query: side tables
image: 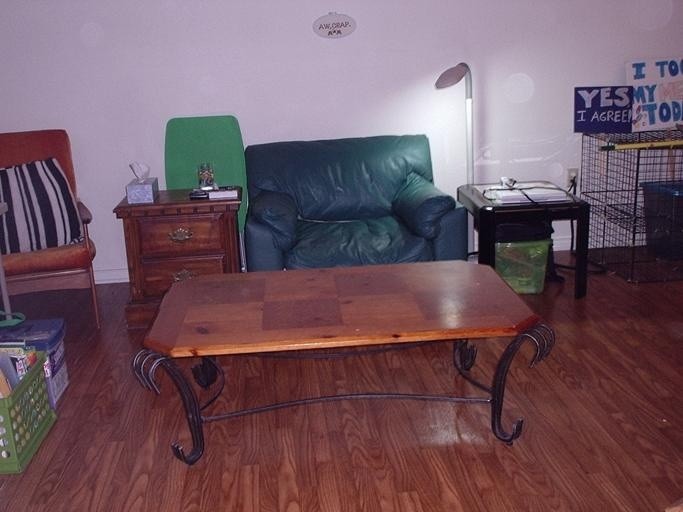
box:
[453,179,592,299]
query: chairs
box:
[243,131,470,271]
[0,128,103,334]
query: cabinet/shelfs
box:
[110,188,242,329]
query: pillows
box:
[0,157,85,255]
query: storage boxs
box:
[494,238,553,295]
[0,315,72,406]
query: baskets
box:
[0,348,58,475]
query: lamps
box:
[434,60,479,262]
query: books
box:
[0,340,50,458]
